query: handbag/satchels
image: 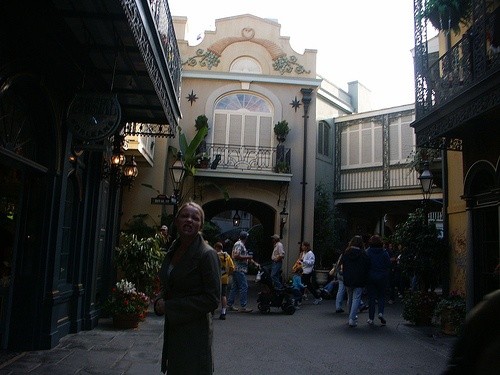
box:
[292,262,303,273]
[329,266,337,276]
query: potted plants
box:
[401,291,437,326]
[437,293,466,336]
[275,162,288,173]
[195,115,209,155]
[273,120,289,166]
[196,152,211,169]
[419,0,473,37]
[113,232,170,321]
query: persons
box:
[271,234,286,288]
[155,202,221,375]
[333,234,404,327]
[299,241,322,305]
[293,267,307,307]
[157,225,262,319]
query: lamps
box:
[122,156,139,193]
[417,161,435,199]
[169,151,189,205]
[233,210,240,227]
[104,145,126,174]
[68,139,89,164]
[279,208,288,225]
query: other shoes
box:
[314,297,322,304]
[220,314,226,320]
[349,319,357,327]
[336,310,344,313]
[367,319,374,324]
[240,307,252,313]
[389,300,395,304]
[399,296,403,299]
[227,305,239,311]
[378,313,386,323]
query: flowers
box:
[106,278,151,330]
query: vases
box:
[315,270,329,288]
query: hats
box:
[271,234,280,241]
[160,225,168,229]
[240,231,249,237]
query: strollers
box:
[255,263,306,315]
